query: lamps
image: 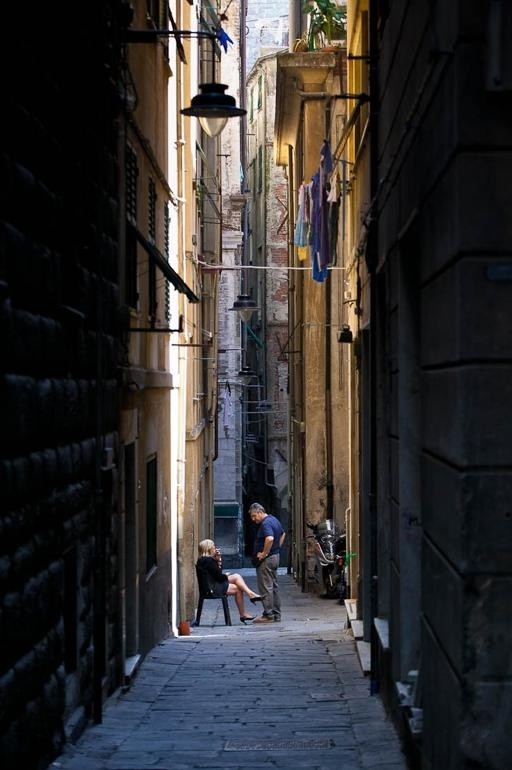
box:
[123,24,248,137]
[197,253,261,322]
[218,346,257,386]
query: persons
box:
[249,503,286,624]
[196,538,266,626]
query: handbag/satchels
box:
[251,557,263,568]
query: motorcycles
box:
[304,515,346,599]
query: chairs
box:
[193,563,232,626]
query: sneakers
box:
[254,616,280,624]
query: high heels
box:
[249,594,266,606]
[240,614,257,625]
